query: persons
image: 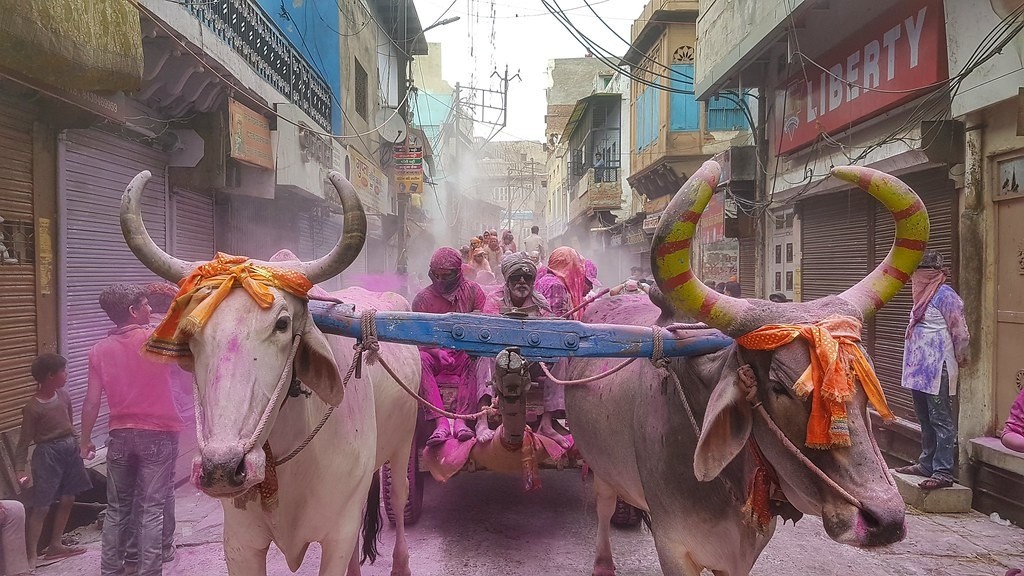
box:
[895,248,973,488]
[459,227,516,282]
[409,248,488,449]
[525,227,547,268]
[80,284,179,576]
[121,285,183,572]
[0,500,35,576]
[533,246,583,377]
[610,265,794,309]
[576,257,603,313]
[14,354,93,576]
[1001,387,1023,451]
[473,252,572,447]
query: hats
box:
[918,249,943,269]
[429,246,463,269]
[470,229,515,264]
[501,251,537,281]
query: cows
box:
[556,157,933,575]
[118,170,418,576]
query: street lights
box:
[399,15,461,130]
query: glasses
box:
[509,274,533,282]
[433,273,453,280]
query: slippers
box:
[427,427,453,446]
[455,427,476,440]
[918,479,953,488]
[896,465,928,476]
[45,545,86,560]
